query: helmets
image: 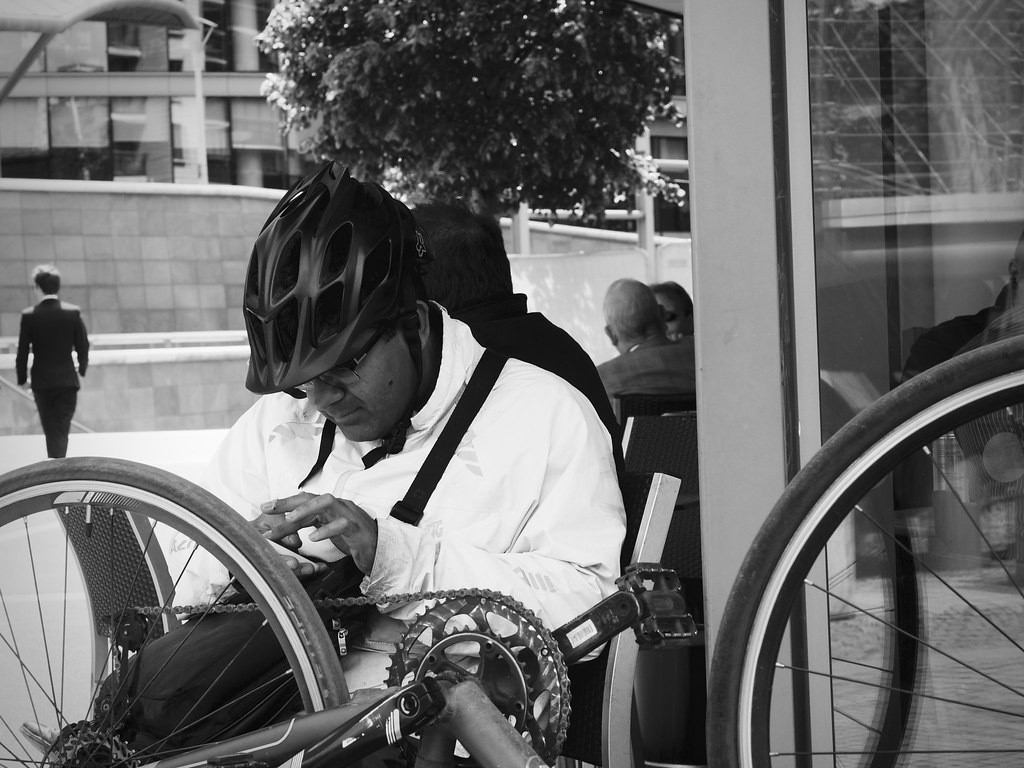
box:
[242,160,434,396]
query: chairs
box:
[53,502,199,723]
[622,414,707,649]
[455,472,684,768]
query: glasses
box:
[297,325,387,389]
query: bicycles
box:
[1,334,1024,768]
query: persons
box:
[410,199,635,577]
[596,278,696,417]
[649,281,694,343]
[900,227,1023,593]
[15,264,90,459]
[184,161,628,768]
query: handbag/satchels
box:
[96,555,377,763]
